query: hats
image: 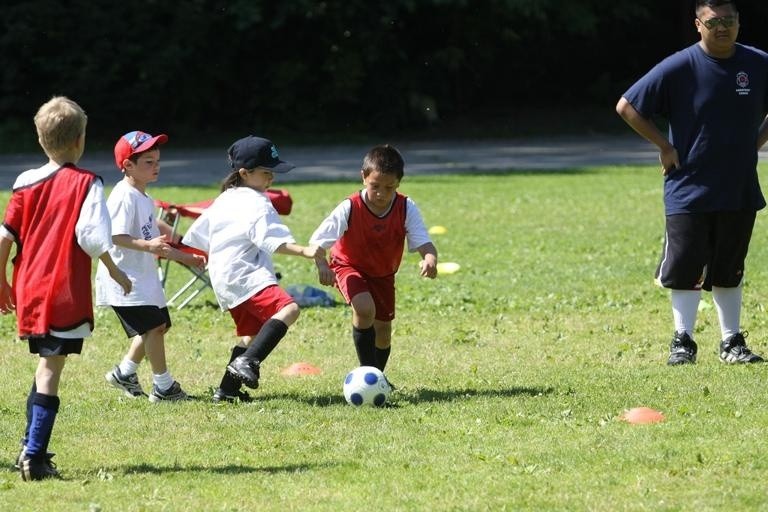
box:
[114,131,168,173]
[228,135,295,173]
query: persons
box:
[181,134,326,404]
[0,94,132,482]
[615,1,768,366]
[96,131,207,404]
[309,144,437,392]
[155,219,187,245]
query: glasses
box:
[697,14,737,28]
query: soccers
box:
[343,366,388,410]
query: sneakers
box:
[20,454,65,481]
[667,331,697,365]
[719,331,763,363]
[105,357,259,403]
